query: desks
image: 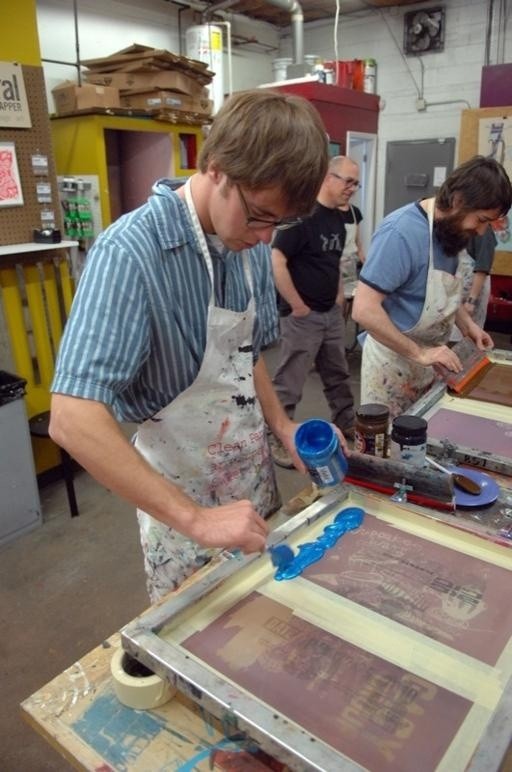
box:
[17,348,511,772]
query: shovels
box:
[424,455,485,495]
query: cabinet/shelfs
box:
[49,110,209,240]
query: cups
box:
[293,418,349,487]
[314,69,334,85]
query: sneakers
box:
[266,422,296,469]
[338,424,357,442]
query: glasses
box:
[332,172,363,191]
[237,189,304,232]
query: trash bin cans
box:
[0,370,44,545]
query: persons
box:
[337,201,365,324]
[47,89,351,603]
[263,154,360,470]
[351,155,512,426]
[450,226,498,347]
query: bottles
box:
[335,58,362,92]
[363,56,377,97]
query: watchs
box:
[466,296,480,307]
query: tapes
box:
[41,229,52,236]
[109,646,178,710]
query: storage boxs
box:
[50,69,216,117]
